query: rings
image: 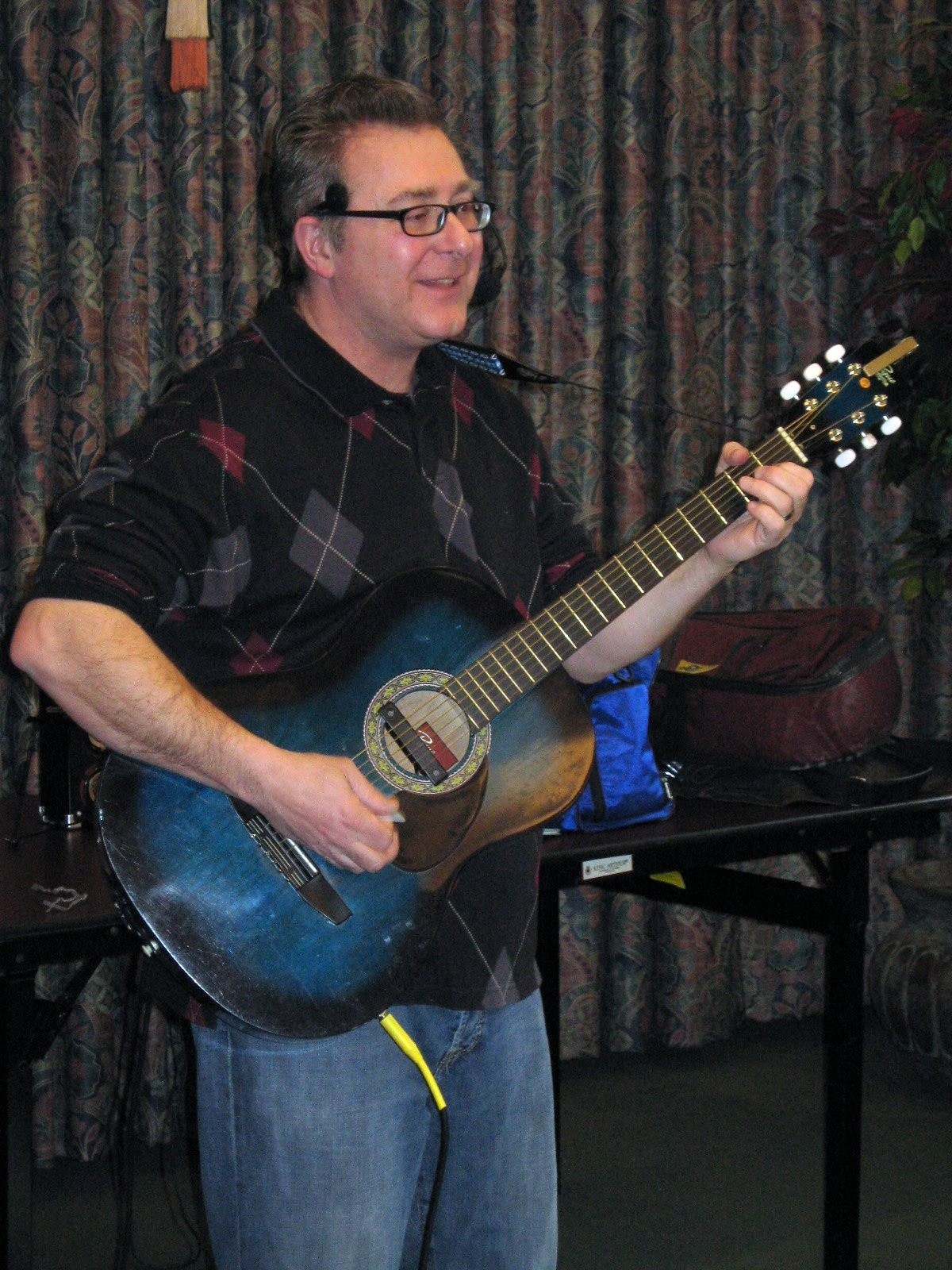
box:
[782,506,795,522]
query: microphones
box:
[475,221,508,303]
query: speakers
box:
[39,696,109,831]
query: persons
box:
[9,73,814,1270]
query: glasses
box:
[307,197,493,237]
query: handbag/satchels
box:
[540,644,675,834]
[647,604,906,776]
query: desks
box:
[0,739,952,1270]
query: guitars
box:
[95,336,938,1045]
